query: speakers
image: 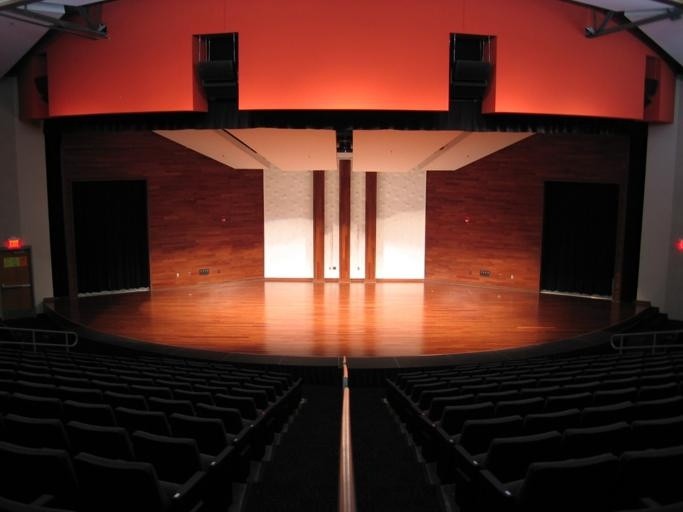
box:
[35,75,48,103]
[645,79,657,97]
[450,81,487,96]
[197,61,235,81]
[453,60,492,81]
[204,83,238,101]
